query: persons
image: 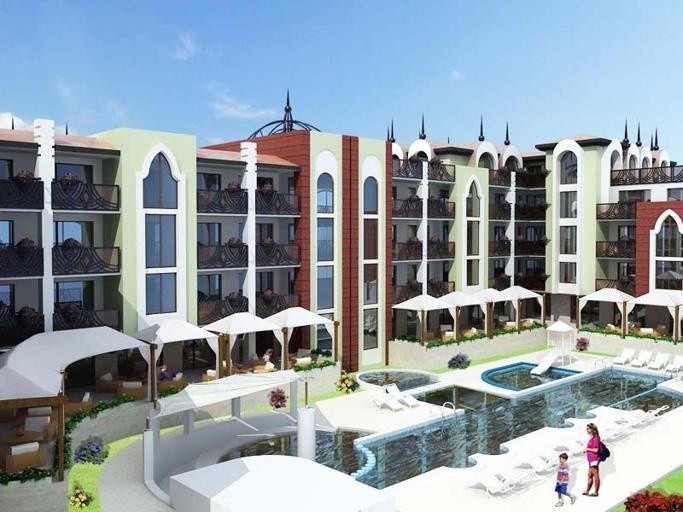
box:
[262,347,274,361]
[582,423,610,496]
[553,452,577,508]
[159,362,182,381]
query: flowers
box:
[266,370,360,410]
[575,335,591,351]
[449,353,471,370]
[68,434,110,508]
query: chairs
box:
[469,404,671,496]
[612,348,682,377]
[157,365,216,391]
[369,382,419,410]
[606,321,667,333]
[223,348,333,374]
[0,407,54,474]
[64,369,148,416]
[439,316,534,344]
